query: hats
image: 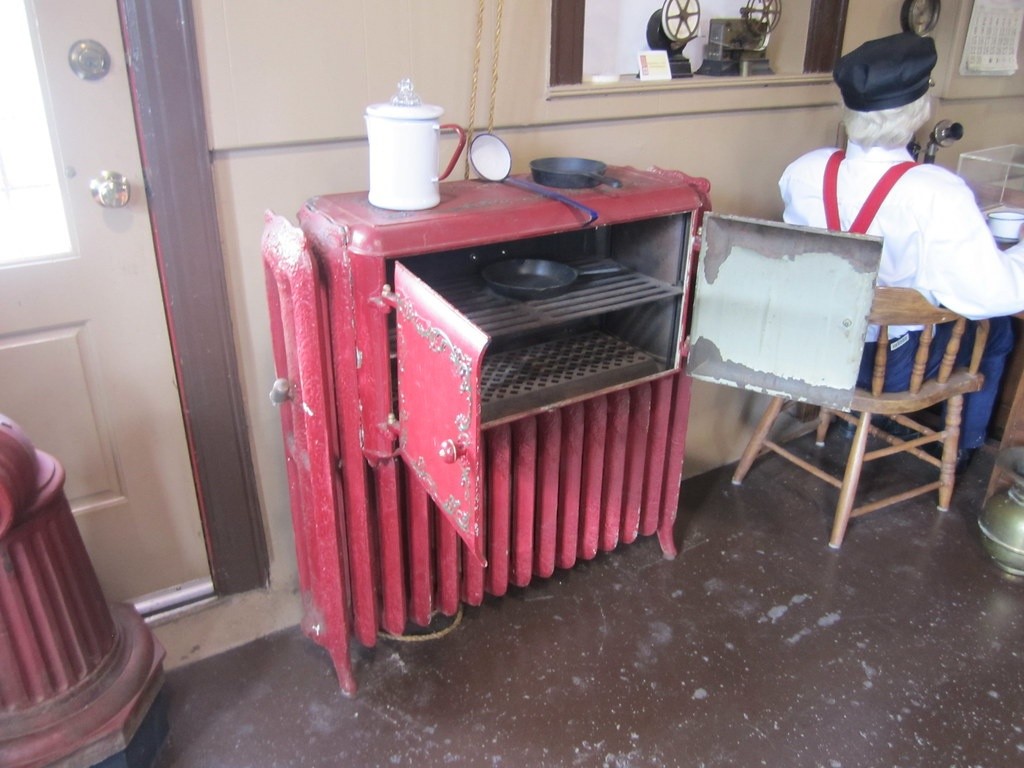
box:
[832,33,938,111]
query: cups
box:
[363,79,467,212]
[987,212,1024,238]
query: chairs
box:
[732,286,991,551]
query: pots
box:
[482,258,632,301]
[528,156,623,189]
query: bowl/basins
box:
[467,133,512,182]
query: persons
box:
[777,30,1024,468]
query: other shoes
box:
[839,413,921,439]
[932,441,975,474]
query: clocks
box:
[901,0,941,35]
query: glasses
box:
[928,78,935,87]
